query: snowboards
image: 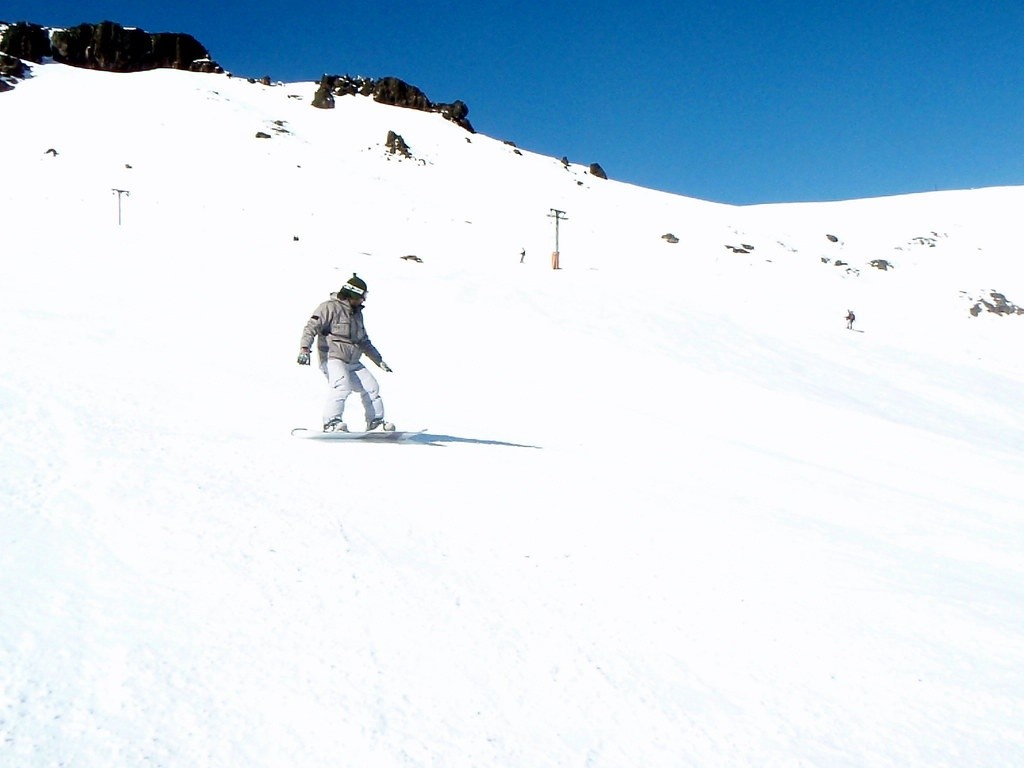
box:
[291,427,428,442]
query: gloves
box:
[379,362,393,373]
[297,352,310,365]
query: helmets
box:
[341,272,367,301]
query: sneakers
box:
[322,420,348,433]
[366,419,396,432]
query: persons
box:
[298,277,395,433]
